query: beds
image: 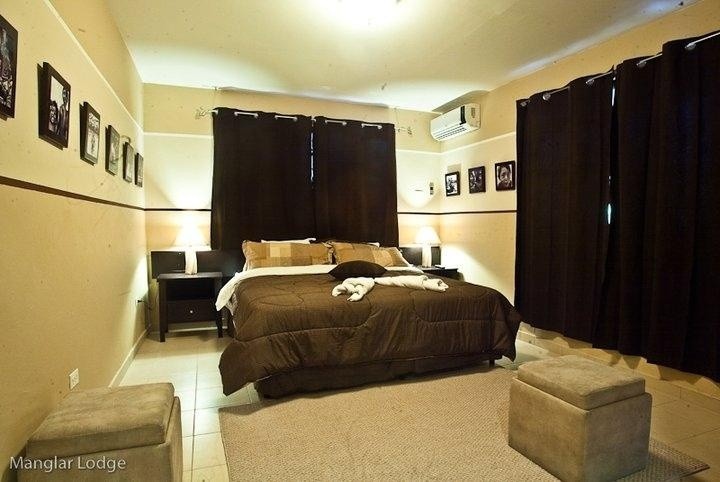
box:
[230,235,522,399]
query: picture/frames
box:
[0,14,19,118]
[37,58,145,187]
[444,170,460,197]
[467,166,487,194]
[493,161,516,191]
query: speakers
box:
[152,251,185,280]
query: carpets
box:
[216,362,708,482]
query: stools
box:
[17,383,186,482]
[505,355,655,482]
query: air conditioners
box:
[429,102,482,142]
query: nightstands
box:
[423,268,460,280]
[157,272,226,344]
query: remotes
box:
[435,264,445,270]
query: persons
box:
[498,164,512,188]
[0,28,15,105]
[49,98,59,133]
[446,177,457,194]
[469,169,483,191]
[58,87,69,139]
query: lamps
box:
[171,221,207,275]
[415,224,442,267]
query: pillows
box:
[240,237,408,273]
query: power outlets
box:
[135,297,143,307]
